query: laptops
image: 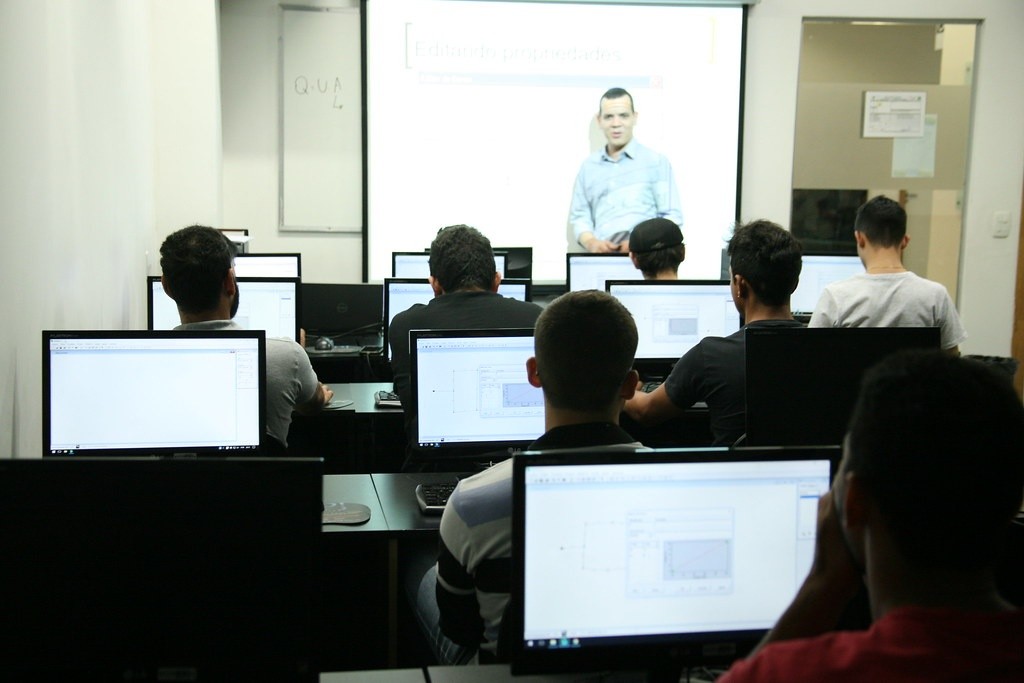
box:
[301,283,384,334]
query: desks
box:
[295,382,645,683]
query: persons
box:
[570,88,682,255]
[712,351,1024,683]
[628,217,686,281]
[399,289,699,666]
[807,195,967,356]
[389,224,544,473]
[159,225,333,455]
[621,222,807,447]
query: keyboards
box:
[415,483,458,514]
[374,391,401,407]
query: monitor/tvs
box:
[0,458,326,683]
[567,253,644,294]
[384,277,531,364]
[792,253,866,322]
[147,275,302,345]
[511,444,844,683]
[43,330,267,458]
[606,280,744,384]
[408,328,545,482]
[745,326,944,445]
[234,253,302,277]
[425,247,533,278]
[392,251,509,278]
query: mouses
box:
[315,336,334,350]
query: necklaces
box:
[868,266,904,269]
